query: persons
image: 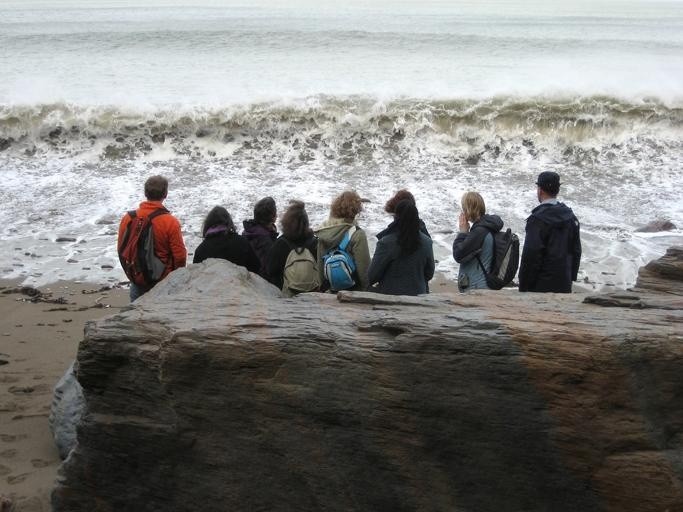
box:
[366,200,434,295]
[381,189,431,290]
[239,195,283,287]
[313,190,371,293]
[452,191,504,293]
[191,205,260,274]
[116,176,186,304]
[262,199,330,292]
[517,171,581,293]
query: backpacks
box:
[470,225,520,290]
[282,236,320,292]
[117,208,170,289]
[321,225,361,293]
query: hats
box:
[535,171,562,185]
